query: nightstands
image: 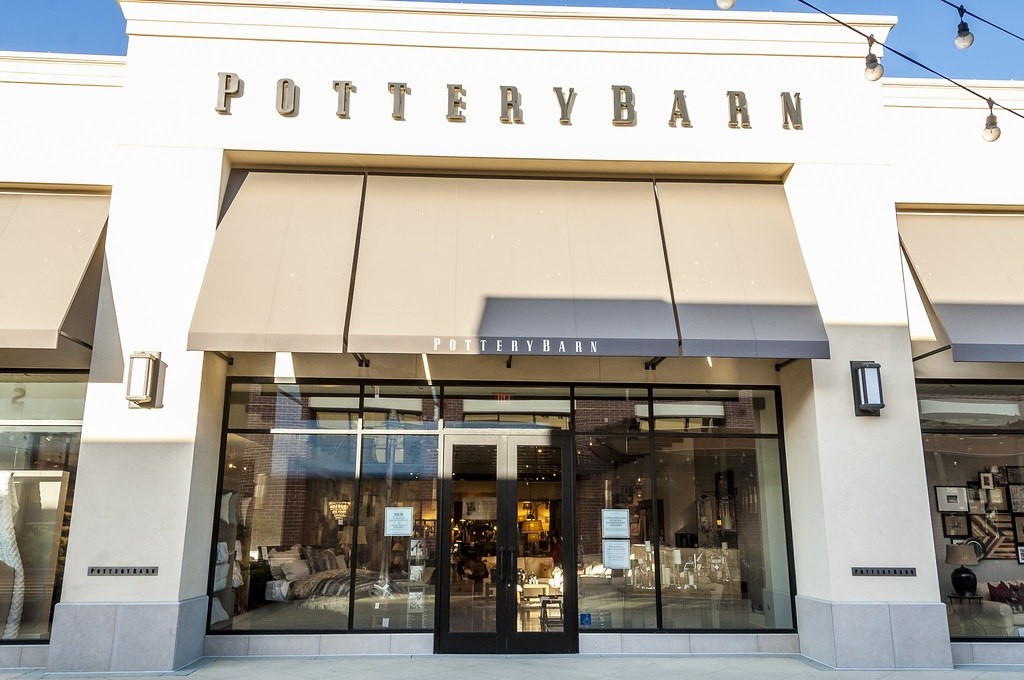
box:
[246,561,270,612]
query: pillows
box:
[220,491,256,527]
[577,560,608,576]
[538,563,554,578]
[267,544,348,583]
[986,581,1024,614]
[211,596,230,626]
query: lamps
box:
[849,360,885,417]
[392,543,405,564]
[339,526,368,544]
[716,493,722,526]
[413,497,551,558]
[623,539,732,592]
[945,544,979,597]
[124,350,162,409]
[329,501,351,526]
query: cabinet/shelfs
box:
[210,476,255,631]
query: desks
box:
[947,594,987,637]
[618,587,751,629]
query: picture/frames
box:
[408,592,423,610]
[601,509,630,538]
[408,538,426,558]
[602,539,631,570]
[933,464,1024,565]
[409,565,424,583]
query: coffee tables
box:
[522,583,549,597]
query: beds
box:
[258,544,409,623]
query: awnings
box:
[187,166,830,374]
[1,191,112,358]
[896,207,1024,368]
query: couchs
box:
[623,544,741,602]
[962,579,1024,638]
[575,552,613,587]
[481,556,564,596]
